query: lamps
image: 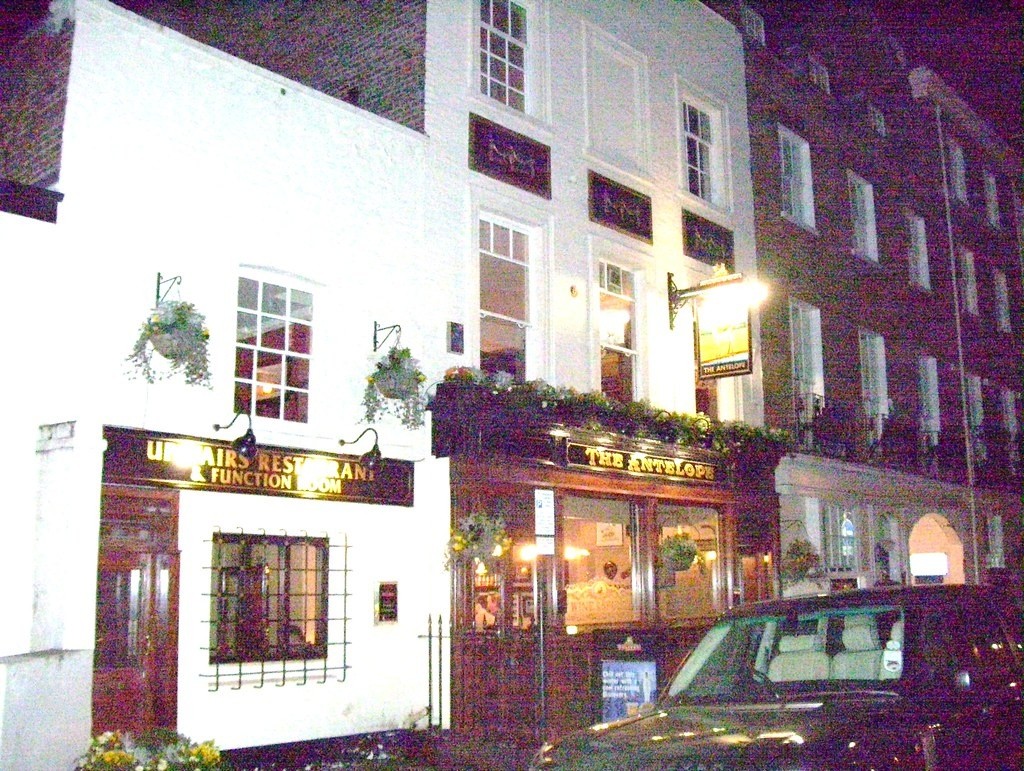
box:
[339,428,382,469]
[213,406,258,458]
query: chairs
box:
[829,613,884,680]
[879,620,904,680]
[767,636,830,680]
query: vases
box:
[150,334,186,359]
[377,380,417,399]
[664,557,692,571]
[458,546,492,559]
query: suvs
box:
[527,585,1023,771]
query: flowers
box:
[431,351,795,457]
[360,346,426,428]
[444,512,512,565]
[73,727,221,771]
[658,534,697,572]
[779,540,818,585]
[124,299,212,389]
